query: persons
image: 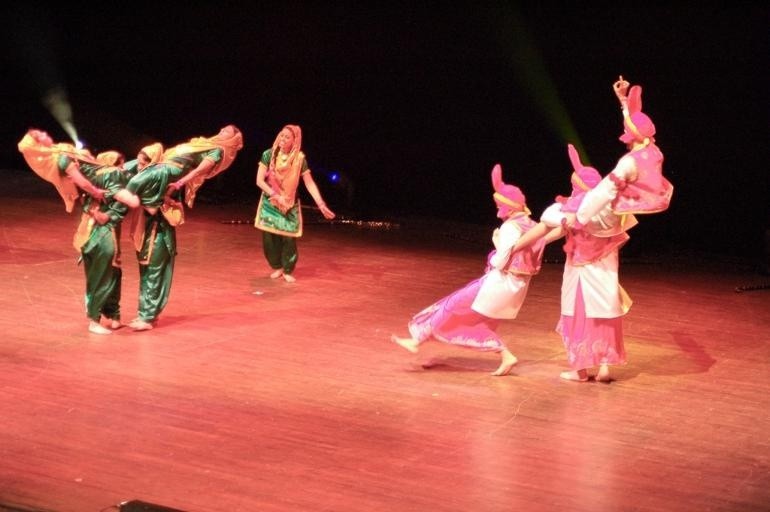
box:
[17,130,160,216]
[393,185,553,376]
[554,166,632,382]
[89,123,244,226]
[126,141,184,331]
[512,112,675,256]
[74,151,129,333]
[253,123,335,283]
